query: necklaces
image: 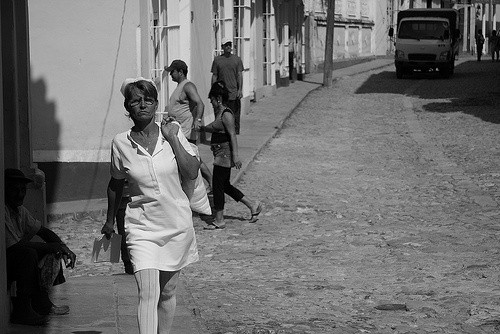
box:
[132,127,156,152]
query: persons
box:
[476,29,485,62]
[161,60,262,230]
[489,30,500,62]
[4,168,76,326]
[210,38,245,134]
[101,77,212,334]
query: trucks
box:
[389,8,460,79]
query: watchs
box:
[195,118,202,121]
